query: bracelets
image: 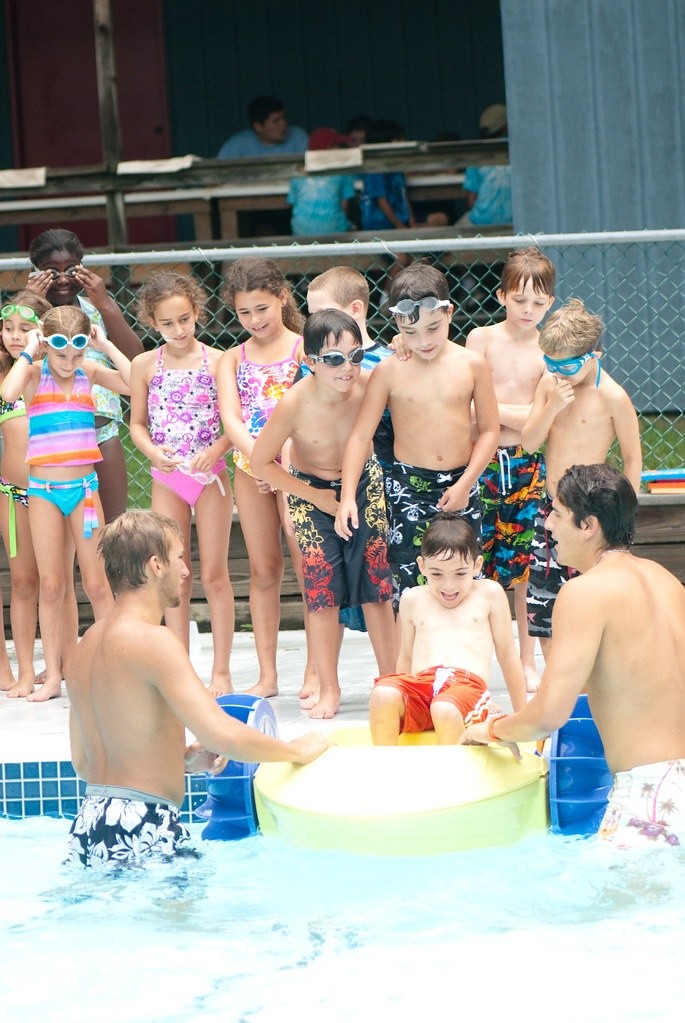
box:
[20,352,32,364]
[488,714,507,741]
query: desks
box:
[0,180,480,304]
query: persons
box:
[217,95,308,238]
[287,128,357,237]
[334,265,499,591]
[65,509,336,875]
[246,308,411,719]
[360,120,449,228]
[465,248,555,693]
[455,104,513,228]
[521,300,643,661]
[217,258,319,700]
[130,271,235,697]
[348,116,371,146]
[1,227,145,703]
[285,265,394,712]
[369,516,526,746]
[458,464,685,844]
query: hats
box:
[308,127,353,149]
[478,103,508,133]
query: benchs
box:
[0,238,513,295]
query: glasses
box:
[27,260,83,282]
[39,332,90,350]
[1,306,39,325]
[389,296,452,315]
[309,346,365,367]
[542,350,584,376]
